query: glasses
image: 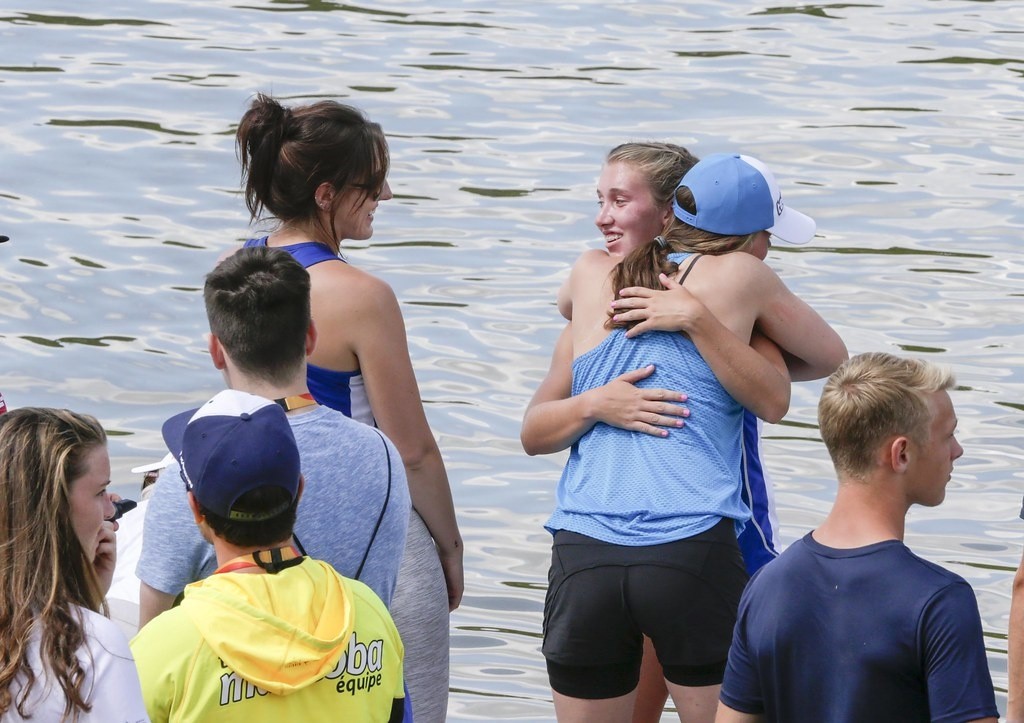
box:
[342,167,387,201]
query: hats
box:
[673,153,816,245]
[162,390,301,520]
[131,452,176,474]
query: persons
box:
[712,352,1001,723]
[135,247,410,632]
[0,406,149,723]
[129,389,408,723]
[520,142,849,722]
[1005,496,1024,723]
[237,95,463,723]
